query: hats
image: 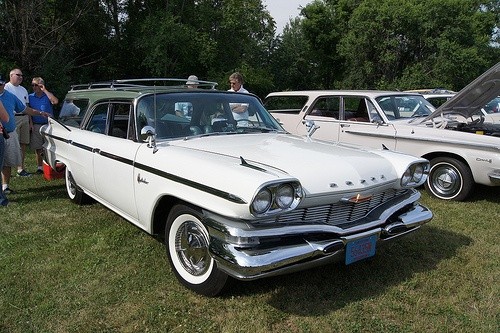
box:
[0,73,6,84]
[185,75,201,84]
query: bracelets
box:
[40,110,44,116]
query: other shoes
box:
[35,169,43,175]
[3,188,16,195]
[16,170,32,176]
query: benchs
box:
[312,111,396,122]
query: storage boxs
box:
[42,159,65,182]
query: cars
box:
[262,61,500,201]
[40,78,434,298]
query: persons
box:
[0,69,58,206]
[174,75,200,121]
[59,97,80,117]
[222,72,250,128]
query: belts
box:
[33,122,47,125]
[15,114,25,116]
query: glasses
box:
[0,84,3,86]
[31,84,39,86]
[16,74,24,77]
[230,82,235,85]
